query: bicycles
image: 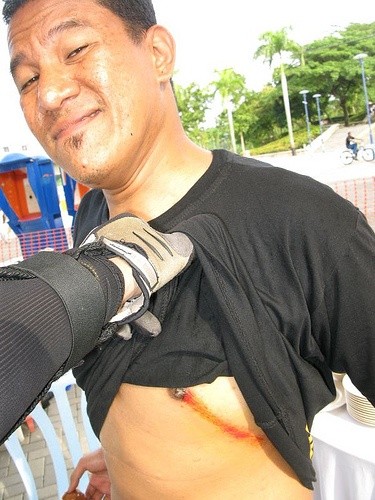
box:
[339,139,375,165]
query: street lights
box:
[352,53,373,144]
[300,90,312,151]
[313,94,324,145]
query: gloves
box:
[79,213,195,341]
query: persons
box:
[0,210,223,447]
[344,130,365,163]
[3,0,375,499]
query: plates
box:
[324,374,375,426]
